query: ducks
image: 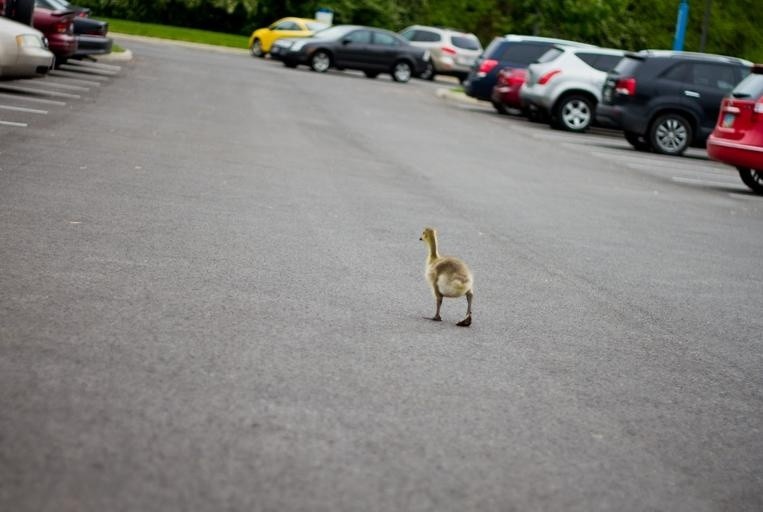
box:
[418,227,473,327]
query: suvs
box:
[525,44,625,132]
[462,34,579,114]
[397,23,483,80]
[596,46,752,155]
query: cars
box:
[248,16,331,57]
[492,66,527,114]
[704,63,763,193]
[269,25,430,83]
[0,0,113,82]
